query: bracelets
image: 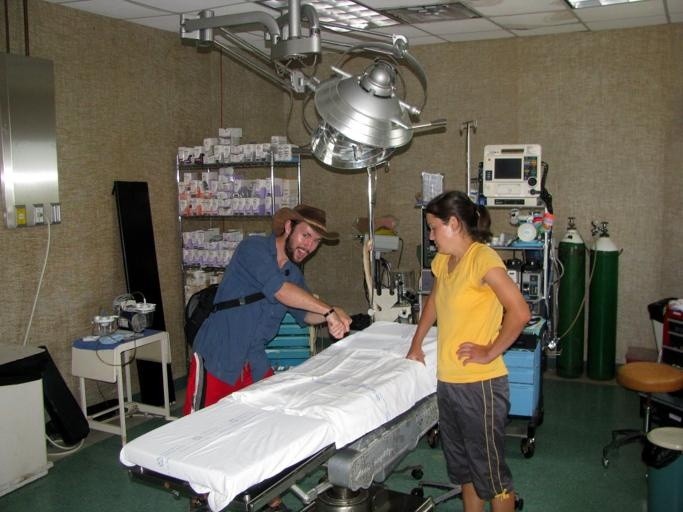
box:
[321,307,336,318]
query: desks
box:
[71,328,179,446]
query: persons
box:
[181,203,354,419]
[402,191,532,511]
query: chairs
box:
[648,297,679,358]
[185,281,220,368]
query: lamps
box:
[179,2,454,179]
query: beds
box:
[120,319,441,511]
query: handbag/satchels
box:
[183,283,218,347]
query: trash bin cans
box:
[646,427,683,512]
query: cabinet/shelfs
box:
[1,52,61,232]
[419,201,556,461]
[0,343,48,499]
[176,140,303,309]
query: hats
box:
[272,205,340,241]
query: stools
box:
[603,360,683,512]
